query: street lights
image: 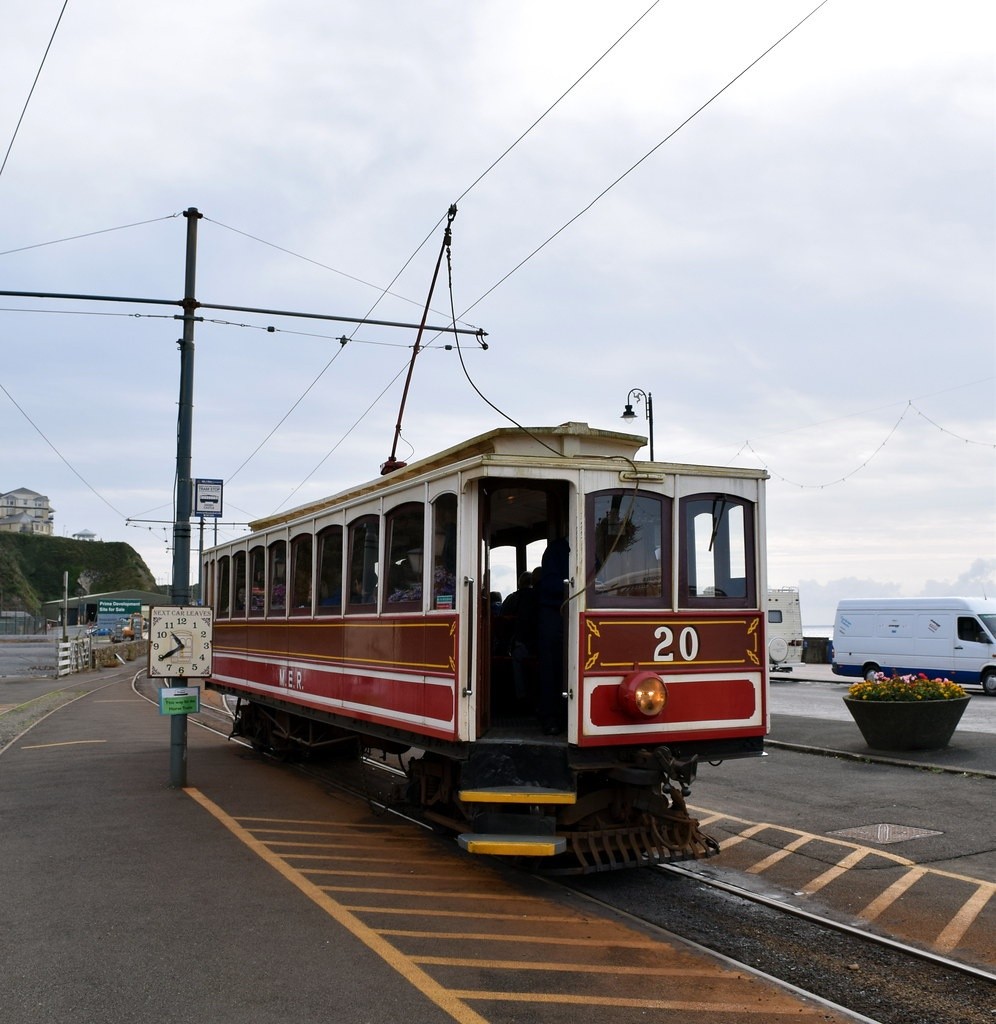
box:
[620,388,654,462]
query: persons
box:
[351,568,378,603]
[489,534,600,734]
[236,586,245,610]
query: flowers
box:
[850,670,967,701]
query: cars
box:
[86,628,114,636]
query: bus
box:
[199,419,771,880]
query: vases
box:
[842,694,974,751]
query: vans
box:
[831,596,996,698]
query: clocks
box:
[148,605,213,678]
